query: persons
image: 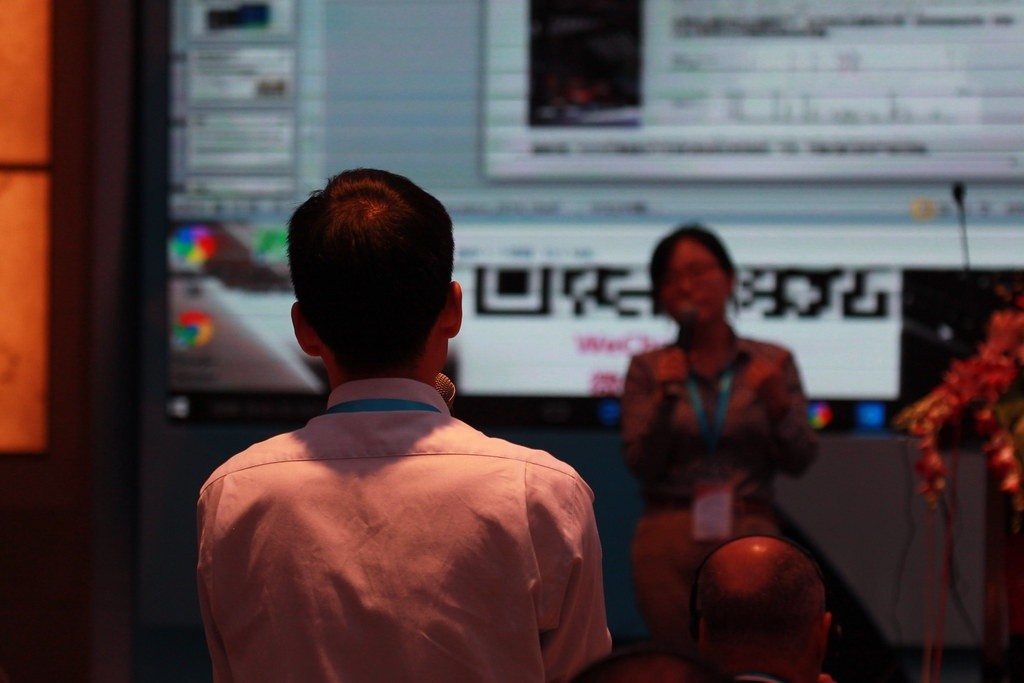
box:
[194,168,839,683]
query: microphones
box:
[434,373,457,416]
[952,182,970,271]
[663,305,698,400]
